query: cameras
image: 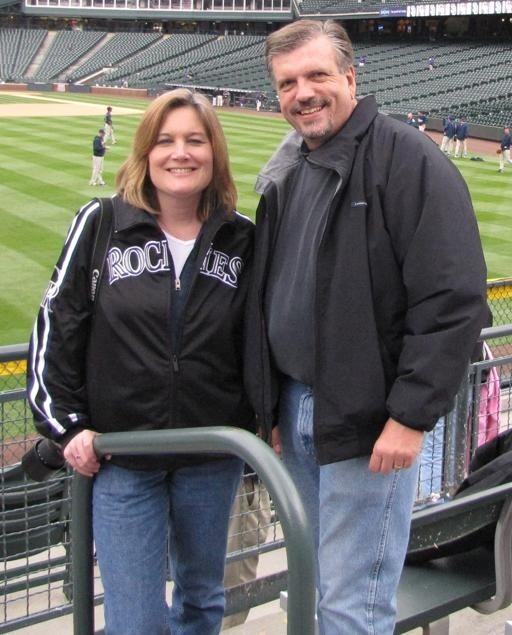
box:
[22,437,66,482]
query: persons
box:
[440,115,455,155]
[253,20,488,635]
[90,129,106,186]
[359,55,367,67]
[417,111,426,132]
[211,88,230,107]
[26,88,258,635]
[454,116,469,158]
[256,90,265,112]
[104,107,117,144]
[122,80,128,88]
[407,112,417,127]
[497,127,512,173]
[427,57,434,70]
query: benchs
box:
[279,482,512,635]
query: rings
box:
[74,455,80,460]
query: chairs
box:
[0,458,75,602]
